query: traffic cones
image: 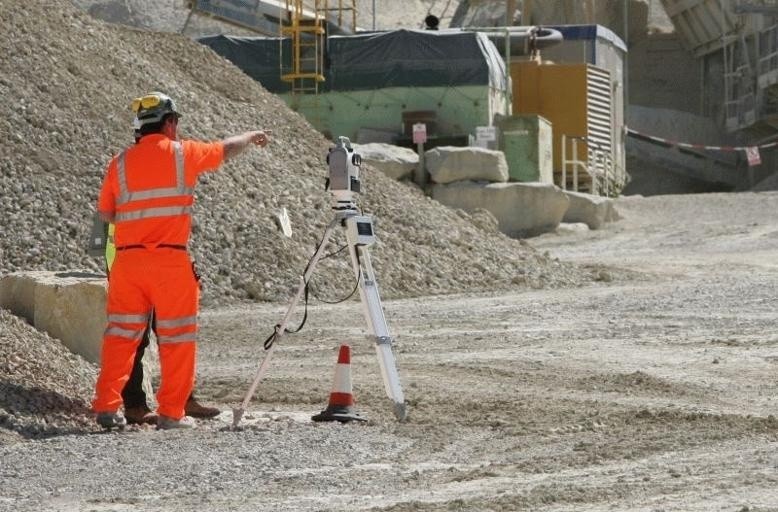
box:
[311,345,370,422]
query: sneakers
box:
[96,397,221,428]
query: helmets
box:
[133,92,182,137]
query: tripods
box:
[231,208,406,428]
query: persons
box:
[100,114,225,429]
[85,89,271,432]
